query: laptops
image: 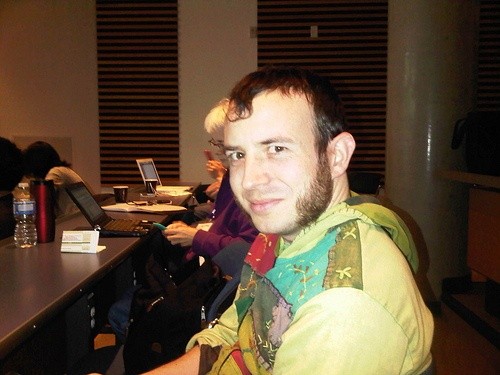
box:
[64,181,154,236]
[136,158,162,188]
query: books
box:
[61,230,106,254]
[102,200,187,213]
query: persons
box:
[22,141,93,217]
[146,65,435,375]
[192,159,229,219]
[161,97,288,258]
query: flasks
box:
[33,179,55,243]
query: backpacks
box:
[123,264,200,375]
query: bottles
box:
[12,183,37,247]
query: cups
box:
[114,186,129,202]
[145,179,158,195]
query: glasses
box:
[207,140,224,152]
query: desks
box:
[0,180,205,375]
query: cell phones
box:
[153,223,168,230]
[204,149,214,161]
